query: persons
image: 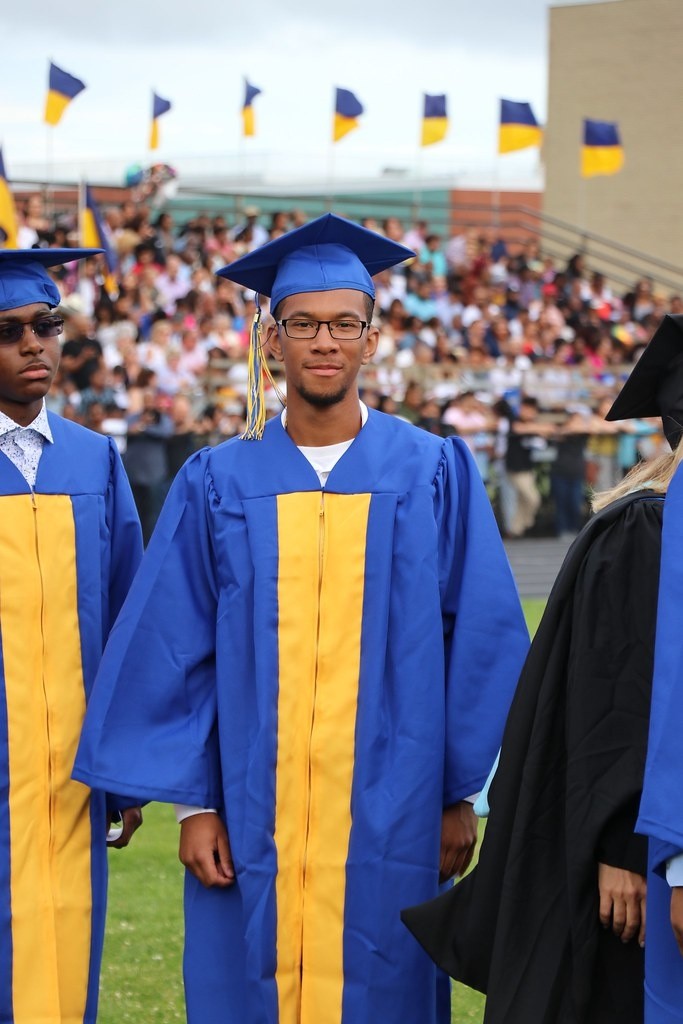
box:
[0,150,683,1024]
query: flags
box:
[0,60,624,301]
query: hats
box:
[1,248,107,311]
[605,314,683,453]
[215,212,417,440]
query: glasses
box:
[0,314,64,343]
[276,318,368,340]
